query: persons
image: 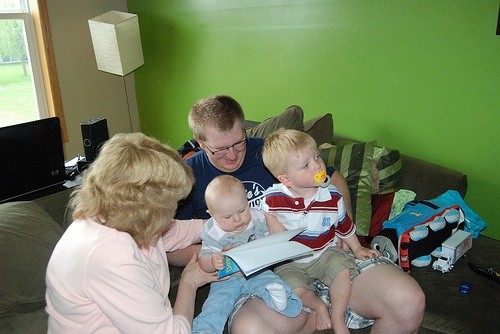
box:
[44,132,286,334]
[259,127,381,334]
[191,174,303,334]
[165,95,425,334]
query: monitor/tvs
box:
[0,117,66,204]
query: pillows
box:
[0,201,65,320]
[244,104,415,252]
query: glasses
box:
[202,132,248,155]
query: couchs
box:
[0,119,500,334]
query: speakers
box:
[79,117,109,161]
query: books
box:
[217,226,314,280]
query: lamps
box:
[88,10,145,133]
